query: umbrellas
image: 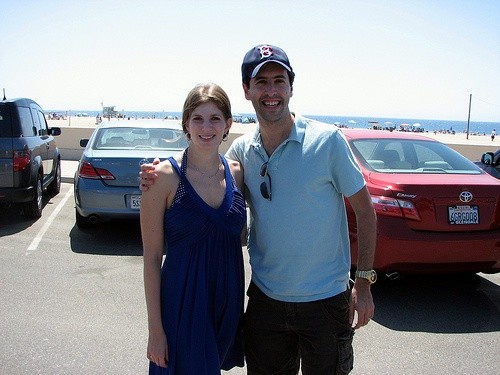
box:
[349,120,420,126]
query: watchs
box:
[356,270,377,284]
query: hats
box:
[241,44,293,80]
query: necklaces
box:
[189,148,221,177]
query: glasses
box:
[260,162,272,201]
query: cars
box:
[73,122,190,231]
[473,149,500,178]
[336,129,500,282]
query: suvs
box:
[0,98,62,219]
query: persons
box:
[491,129,496,141]
[140,44,377,375]
[140,83,247,375]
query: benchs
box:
[106,136,178,148]
[366,159,448,170]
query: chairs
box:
[379,149,400,166]
